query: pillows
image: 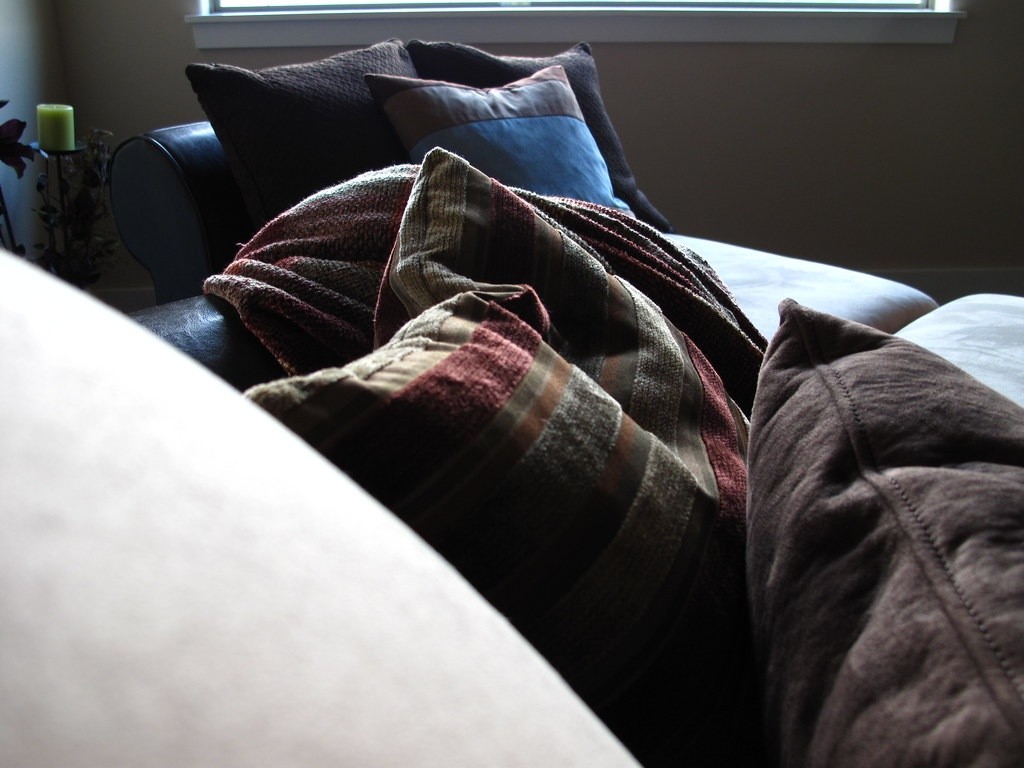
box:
[238,148,749,729]
[183,37,420,234]
[203,164,768,416]
[746,300,1024,768]
[363,65,635,221]
[406,36,673,235]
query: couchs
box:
[131,120,1024,414]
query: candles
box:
[36,103,75,153]
